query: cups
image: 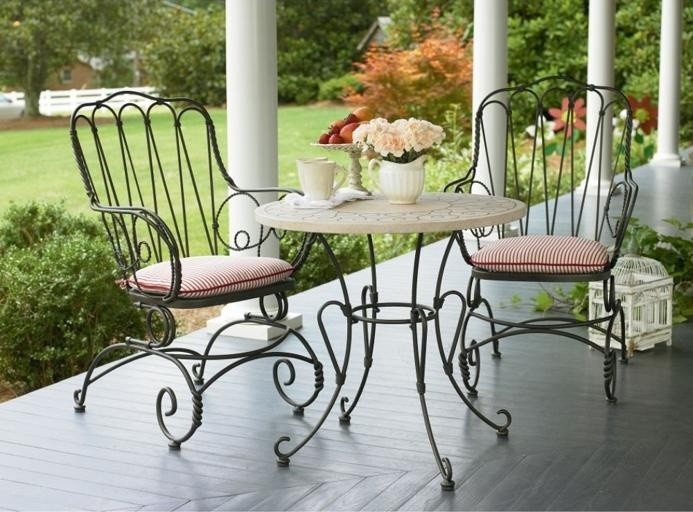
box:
[296,155,349,203]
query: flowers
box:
[351,116,448,155]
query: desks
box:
[252,190,529,493]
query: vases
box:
[367,153,430,205]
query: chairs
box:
[443,74,639,405]
[70,89,325,452]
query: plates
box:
[291,195,345,208]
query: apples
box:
[331,107,373,143]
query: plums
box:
[319,125,343,144]
